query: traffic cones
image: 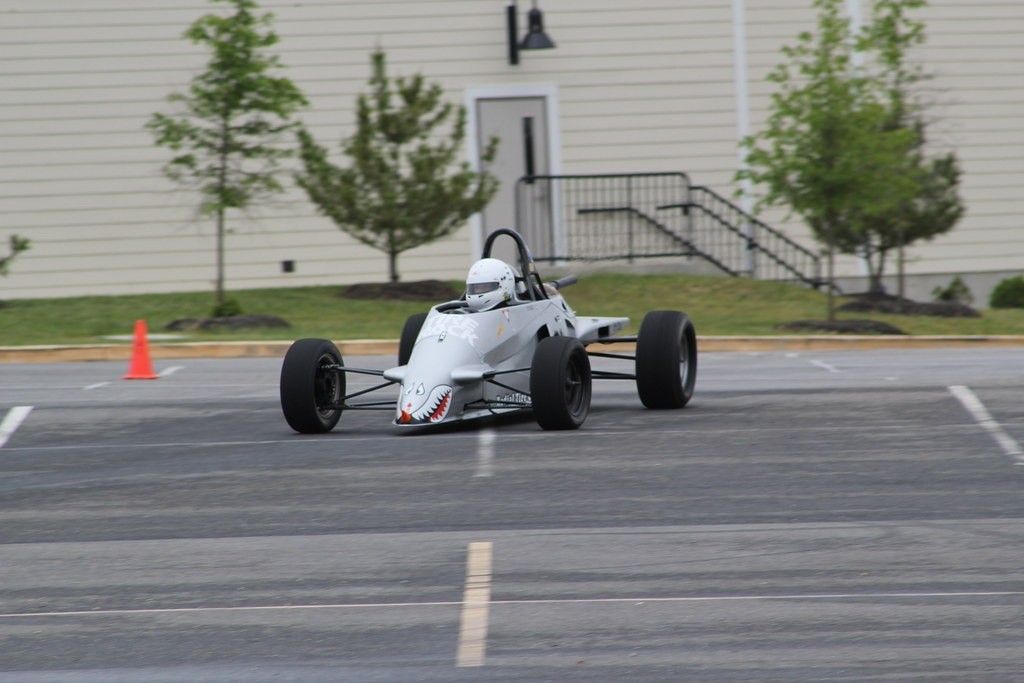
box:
[118,320,160,380]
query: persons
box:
[465,259,514,311]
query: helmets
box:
[466,259,515,311]
